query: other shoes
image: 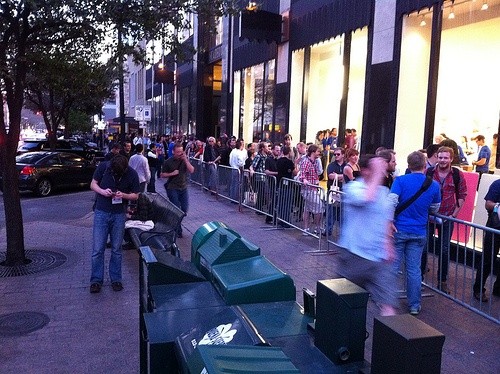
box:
[107,242,111,247]
[411,305,421,315]
[437,282,450,295]
[314,226,325,234]
[90,283,101,292]
[175,228,183,238]
[256,211,266,215]
[474,291,488,302]
[303,228,310,236]
[122,242,136,250]
[266,220,274,224]
[113,281,123,291]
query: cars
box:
[0,130,106,199]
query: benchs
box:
[127,191,186,257]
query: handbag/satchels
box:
[329,174,341,207]
[243,191,257,206]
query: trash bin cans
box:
[197,227,260,284]
[212,256,296,305]
[187,345,299,374]
[192,221,241,270]
[174,306,271,374]
[150,281,227,312]
[137,246,207,374]
[142,308,225,374]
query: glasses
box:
[334,153,342,156]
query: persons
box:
[473,179,500,301]
[404,133,469,174]
[96,128,395,236]
[488,134,498,174]
[161,143,194,238]
[333,153,399,316]
[389,151,440,314]
[472,135,491,191]
[90,155,140,294]
[421,146,467,295]
